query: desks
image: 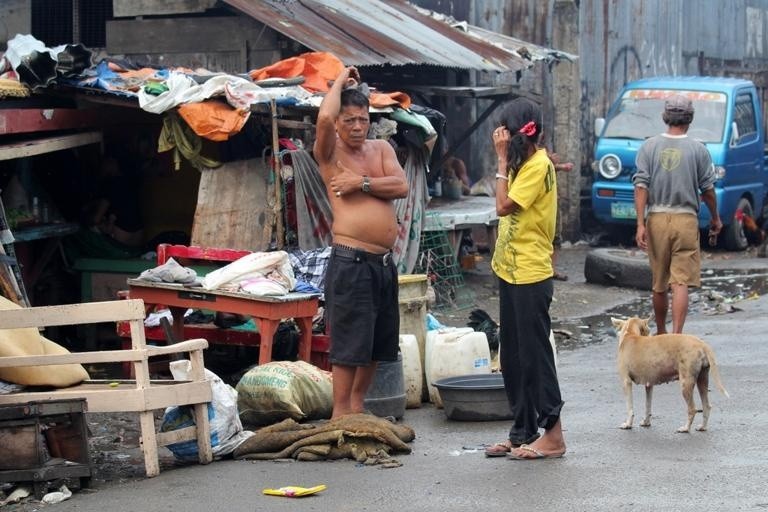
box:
[422,196,500,257]
[126,278,321,373]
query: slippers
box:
[485,443,510,457]
[504,443,561,461]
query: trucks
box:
[593,76,767,250]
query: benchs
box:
[0,299,214,476]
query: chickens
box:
[464,308,500,354]
[733,208,767,258]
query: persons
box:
[483,98,568,463]
[310,64,410,422]
[538,144,574,282]
[631,94,722,335]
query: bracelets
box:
[494,173,511,183]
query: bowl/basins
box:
[431,373,516,421]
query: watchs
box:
[362,176,370,193]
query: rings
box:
[336,192,342,196]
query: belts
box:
[330,247,392,267]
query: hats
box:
[663,95,693,114]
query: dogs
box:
[610,312,732,433]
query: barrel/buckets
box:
[434,330,492,407]
[398,332,426,408]
[364,350,407,422]
[423,326,472,402]
